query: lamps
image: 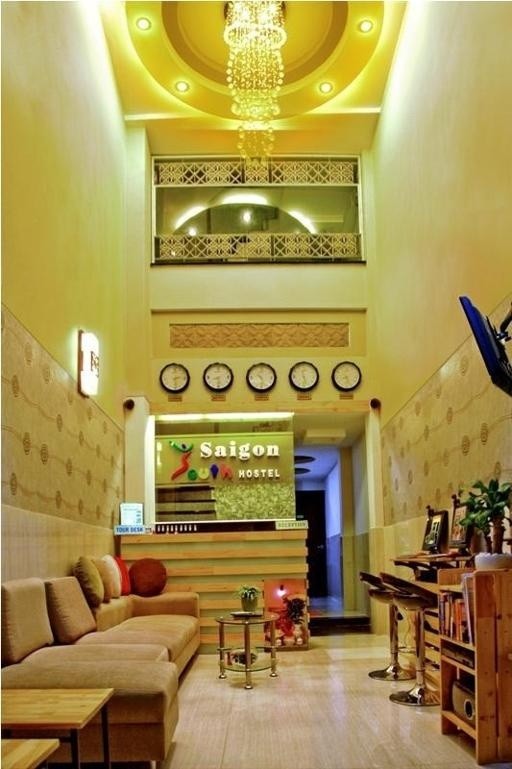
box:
[77,329,100,398]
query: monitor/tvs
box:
[458,296,512,396]
[422,509,446,553]
[450,503,471,553]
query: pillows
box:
[74,556,103,613]
[45,574,96,644]
[128,558,166,596]
[114,555,129,599]
[100,553,120,598]
[92,556,114,608]
[3,576,54,665]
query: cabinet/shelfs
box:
[435,569,511,764]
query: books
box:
[440,574,473,643]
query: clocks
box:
[158,362,190,394]
[330,360,362,392]
[288,361,320,392]
[203,361,233,391]
[246,363,277,393]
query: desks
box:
[2,738,62,769]
[2,687,120,767]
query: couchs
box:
[2,554,203,769]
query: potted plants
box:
[236,582,263,613]
[287,594,310,648]
[453,477,512,569]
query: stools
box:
[380,571,450,709]
[360,570,419,682]
